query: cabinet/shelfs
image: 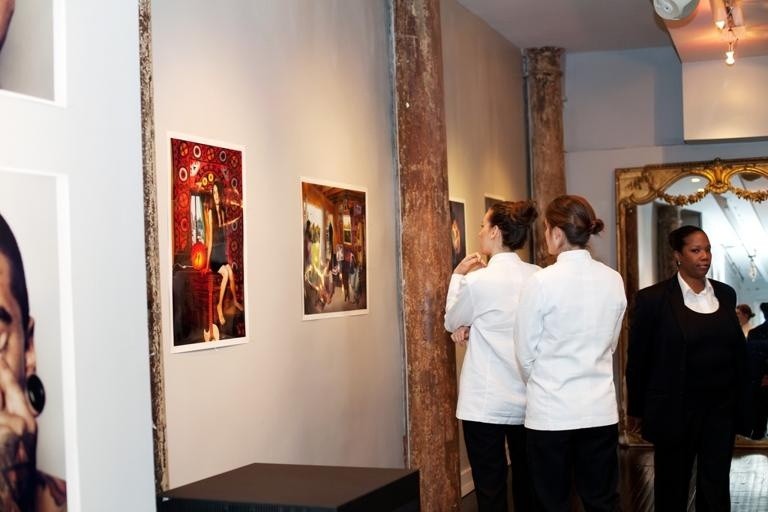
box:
[159,461,422,510]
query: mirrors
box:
[612,156,768,448]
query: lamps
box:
[708,1,744,65]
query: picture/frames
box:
[0,0,69,114]
[166,132,250,353]
[484,193,506,267]
[299,176,371,320]
[0,166,86,511]
[449,197,467,263]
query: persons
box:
[748,302,768,440]
[451,212,465,267]
[628,223,768,512]
[0,214,66,512]
[445,198,544,512]
[206,181,244,325]
[736,304,756,341]
[513,193,629,512]
